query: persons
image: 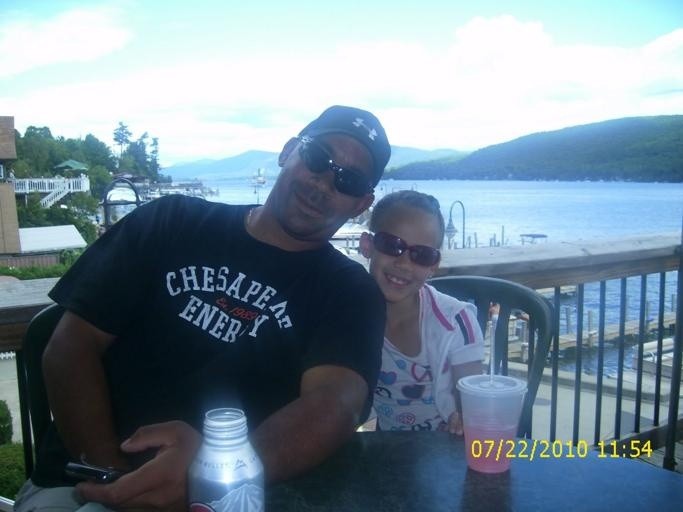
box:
[359,190,486,434]
[42,104,391,512]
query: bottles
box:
[185,406,265,512]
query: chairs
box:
[424,273,557,443]
[18,298,116,487]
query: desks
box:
[266,428,682,512]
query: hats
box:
[299,105,392,188]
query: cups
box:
[455,372,529,474]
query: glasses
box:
[298,136,374,197]
[368,231,441,267]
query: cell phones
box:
[66,463,129,483]
